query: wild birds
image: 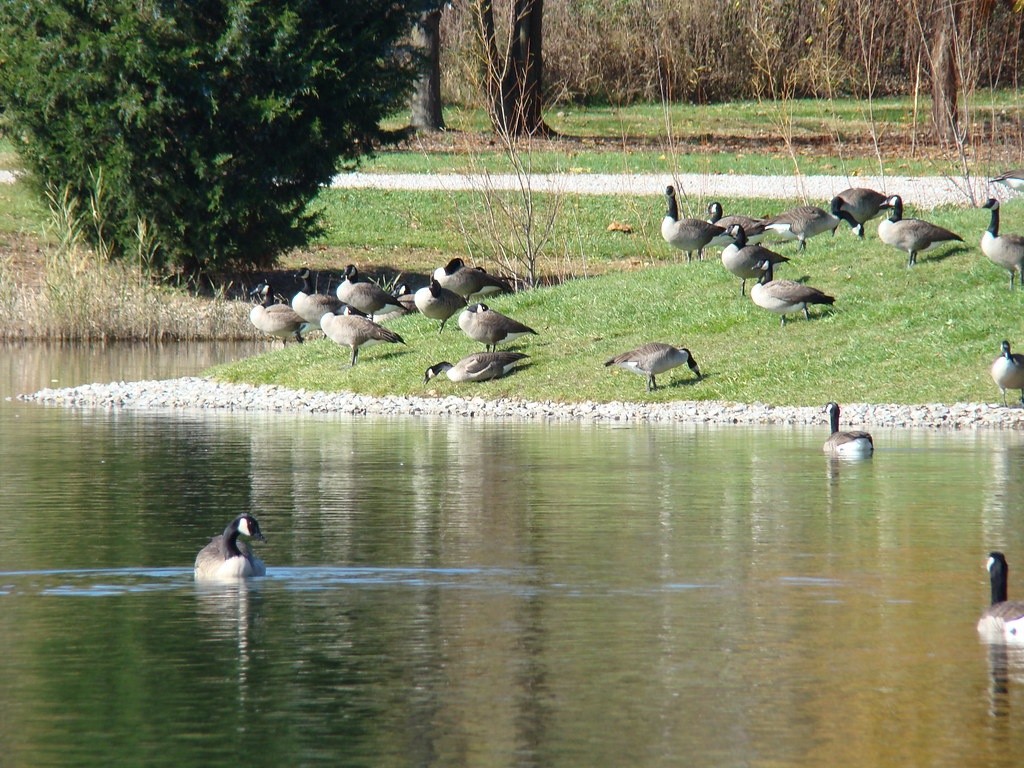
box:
[980,168,1024,289]
[193,513,268,578]
[989,341,1024,409]
[977,551,1024,643]
[603,341,702,391]
[661,186,965,328]
[821,402,874,454]
[249,257,541,385]
